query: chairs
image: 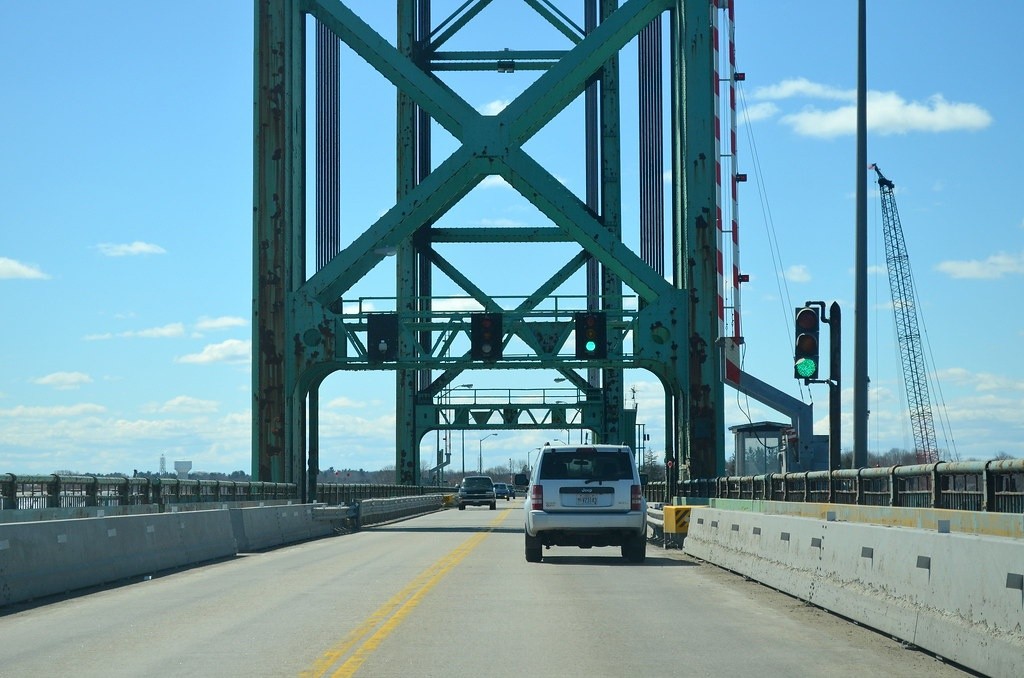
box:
[551,464,568,476]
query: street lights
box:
[554,377,580,409]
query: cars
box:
[454,476,497,510]
[494,483,510,501]
[523,445,649,563]
[507,484,516,499]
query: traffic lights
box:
[795,307,818,380]
[479,434,498,476]
[527,447,541,471]
[471,314,503,360]
[435,383,473,485]
[577,314,605,359]
